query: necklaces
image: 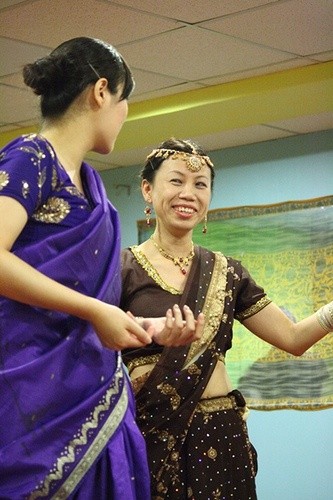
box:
[149,236,195,277]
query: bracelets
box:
[315,303,333,335]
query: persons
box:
[109,137,333,500]
[0,36,207,500]
